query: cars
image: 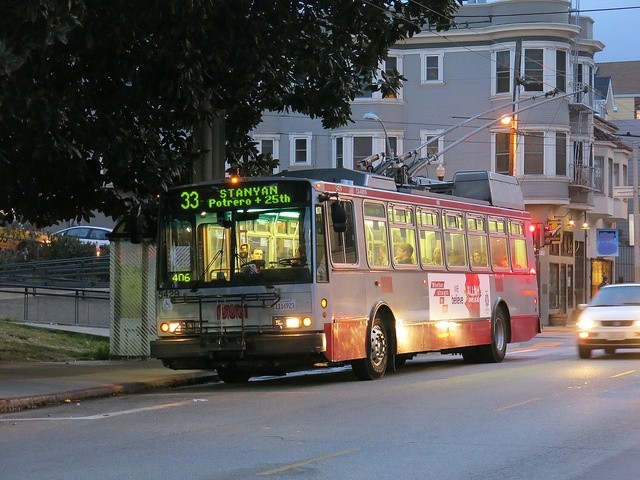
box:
[48,226,114,249]
[575,284,640,359]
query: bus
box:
[150,169,543,384]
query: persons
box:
[619,278,623,283]
[397,244,413,264]
[501,257,508,267]
[430,248,441,265]
[472,250,486,267]
[240,244,249,265]
[448,251,464,266]
[251,248,264,260]
[481,253,487,265]
[598,275,608,289]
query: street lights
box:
[363,112,392,177]
